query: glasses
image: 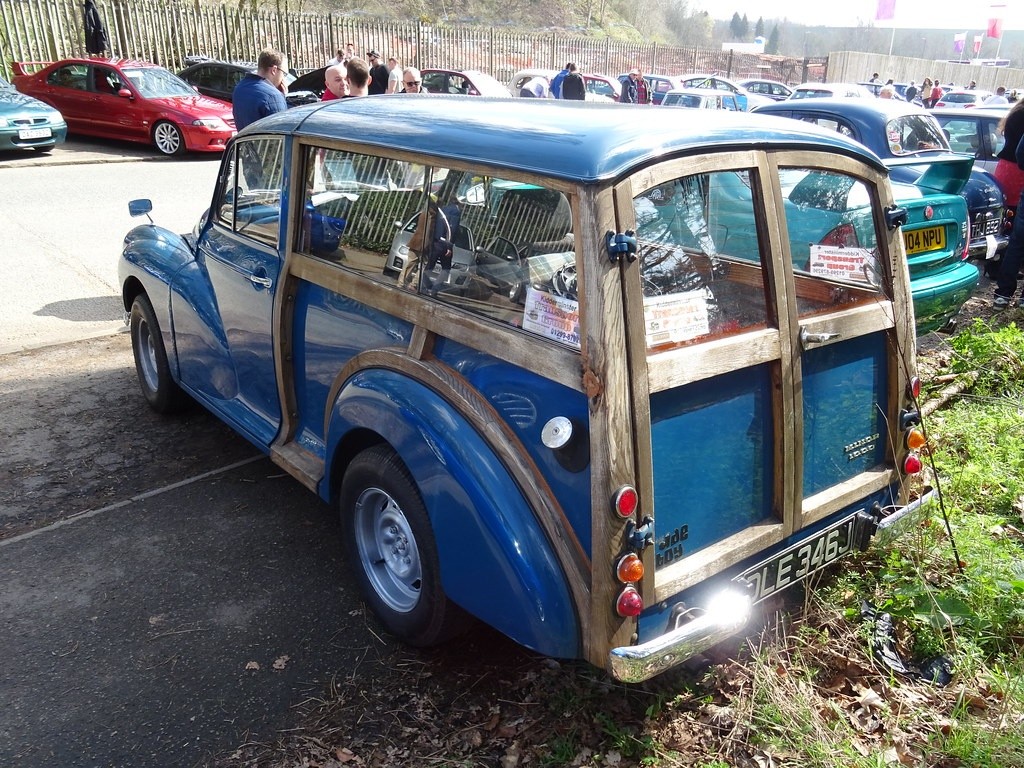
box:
[369,58,376,62]
[405,81,420,87]
[269,66,288,76]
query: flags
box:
[874,0,896,20]
[953,19,1002,54]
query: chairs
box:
[95,69,117,93]
[966,130,998,159]
[344,187,427,257]
[487,186,577,263]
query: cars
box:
[175,61,334,109]
[579,74,622,101]
[660,88,741,111]
[507,69,561,99]
[936,91,993,108]
[676,75,776,112]
[747,98,1012,263]
[11,58,238,156]
[419,69,515,98]
[926,109,1022,207]
[0,76,67,156]
[633,168,981,313]
[117,94,940,684]
[861,83,959,106]
[735,78,793,100]
[788,83,875,101]
[618,74,716,105]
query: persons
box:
[520,75,551,98]
[928,79,943,107]
[232,47,288,190]
[400,67,442,187]
[551,62,586,100]
[904,81,918,103]
[318,66,351,189]
[1008,89,1019,102]
[964,80,976,89]
[997,87,1007,96]
[367,51,389,95]
[388,58,403,94]
[326,50,344,66]
[920,77,933,107]
[322,58,406,189]
[866,74,879,94]
[636,69,652,103]
[879,79,895,100]
[994,98,1024,217]
[394,196,465,296]
[345,43,355,59]
[619,69,638,103]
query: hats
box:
[629,69,639,75]
[367,51,380,58]
